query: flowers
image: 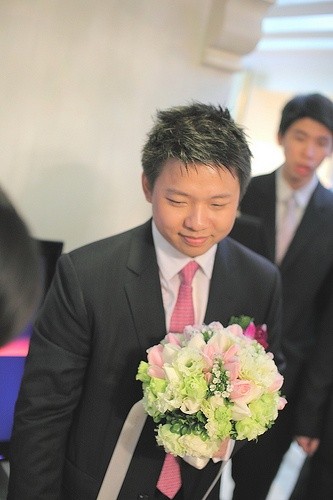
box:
[137,315,287,460]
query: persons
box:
[1,190,43,346]
[228,94,333,500]
[290,269,333,500]
[6,104,282,500]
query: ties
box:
[274,198,296,265]
[157,262,199,499]
